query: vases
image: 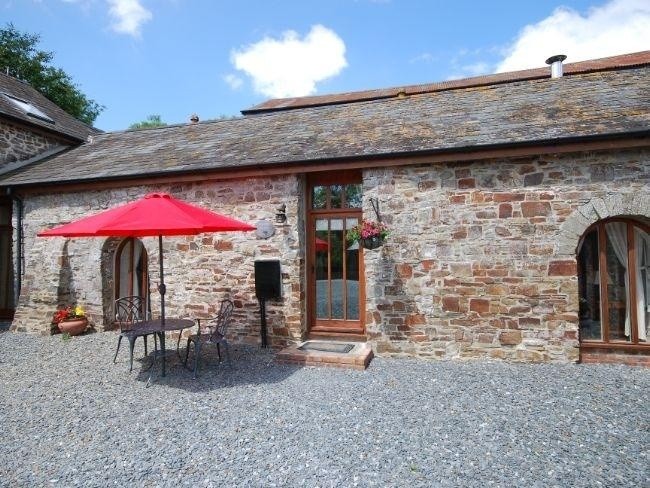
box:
[58,317,88,335]
[358,237,382,249]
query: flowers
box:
[344,220,390,240]
[50,307,92,334]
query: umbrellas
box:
[35,191,259,376]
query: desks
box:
[132,319,194,377]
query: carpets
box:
[296,341,355,354]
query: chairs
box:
[185,300,234,380]
[113,296,156,371]
